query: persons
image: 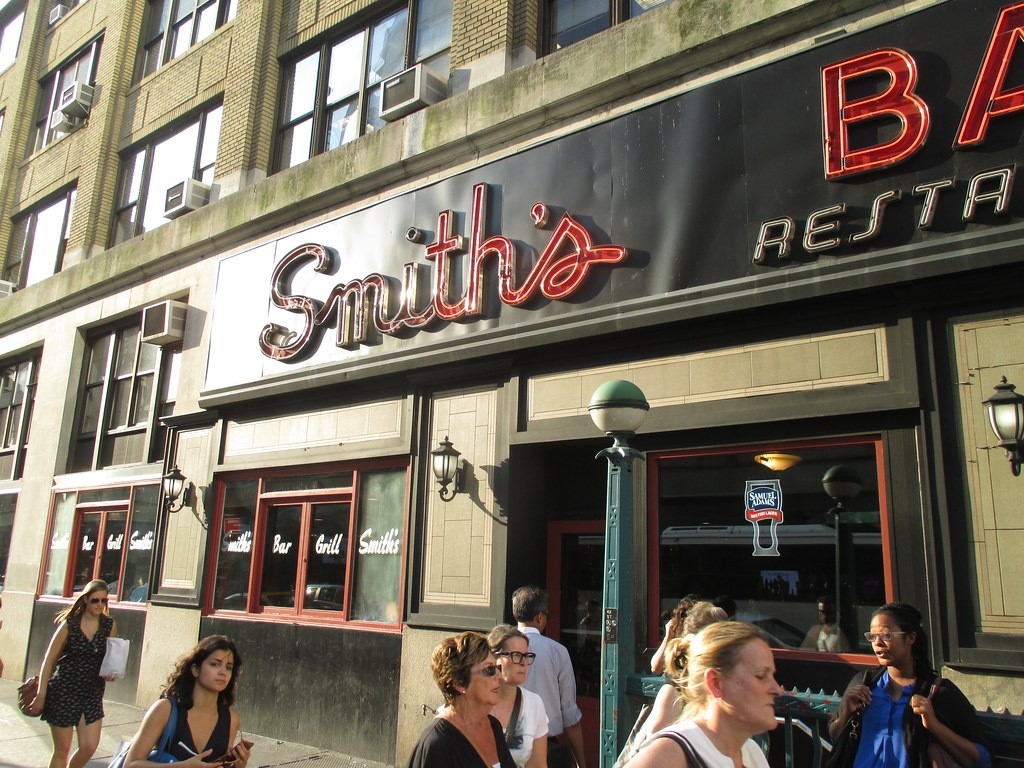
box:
[489,625,549,768]
[406,631,518,768]
[512,585,587,768]
[621,595,783,768]
[123,634,249,768]
[830,603,993,768]
[816,597,850,653]
[28,578,117,768]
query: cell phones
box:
[214,741,253,764]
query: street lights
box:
[589,381,650,768]
[822,466,863,653]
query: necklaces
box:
[93,641,97,652]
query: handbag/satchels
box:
[18,676,44,716]
[924,674,973,768]
[108,697,178,768]
[826,668,871,768]
[611,681,684,768]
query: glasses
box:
[864,631,912,641]
[496,651,537,666]
[468,664,503,677]
[90,598,110,604]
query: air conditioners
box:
[379,62,450,122]
[139,298,187,344]
[48,4,70,25]
[0,278,13,298]
[57,80,94,118]
[51,106,80,133]
[163,177,211,220]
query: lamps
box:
[430,435,466,502]
[161,464,192,513]
[981,372,1023,478]
[754,452,805,475]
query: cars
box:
[225,583,344,611]
